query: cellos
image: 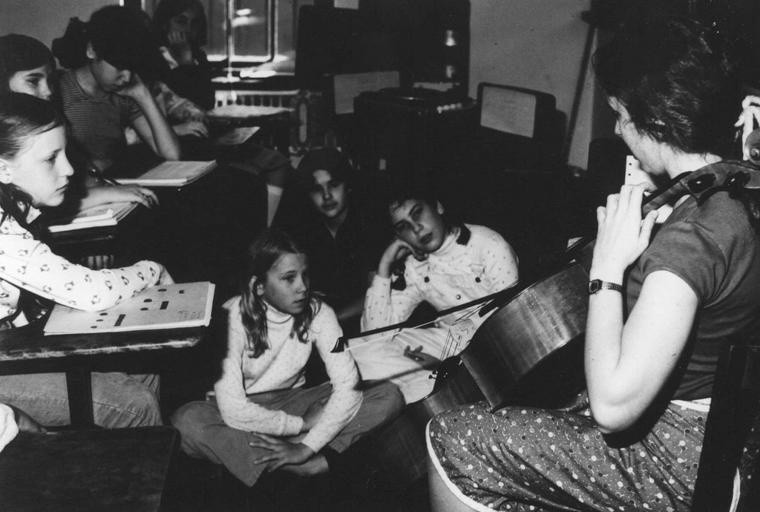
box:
[330,129,759,512]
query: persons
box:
[423,0,759,511]
[175,148,518,485]
[0,2,292,429]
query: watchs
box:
[587,279,623,294]
